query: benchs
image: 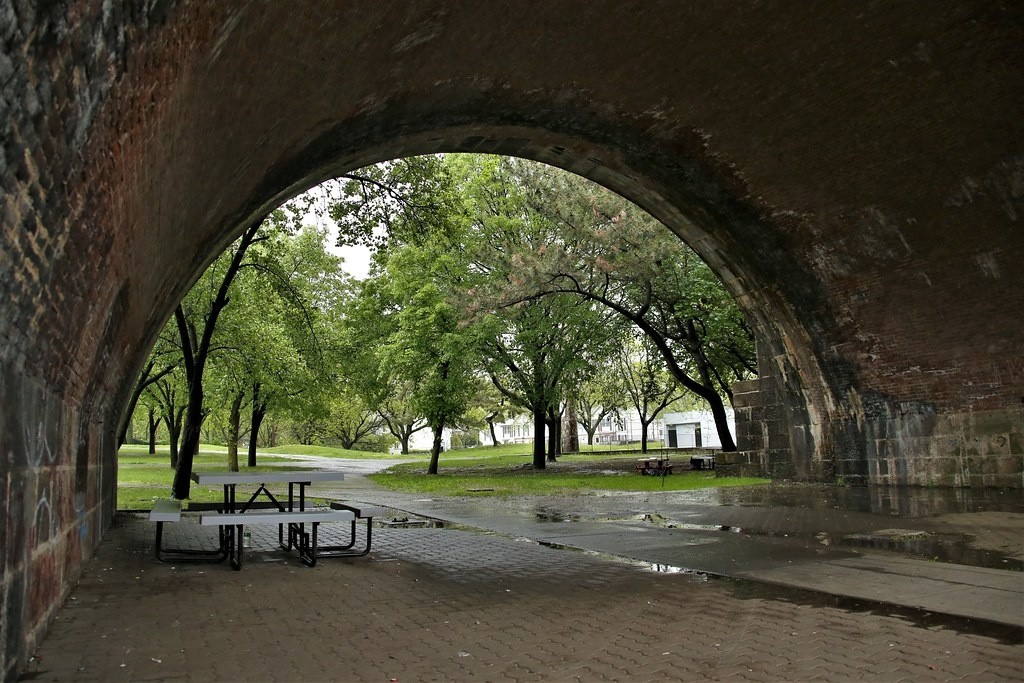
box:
[150,498,386,571]
[638,464,673,474]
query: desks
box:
[638,457,669,474]
[692,454,714,470]
[704,448,722,468]
[191,471,345,564]
